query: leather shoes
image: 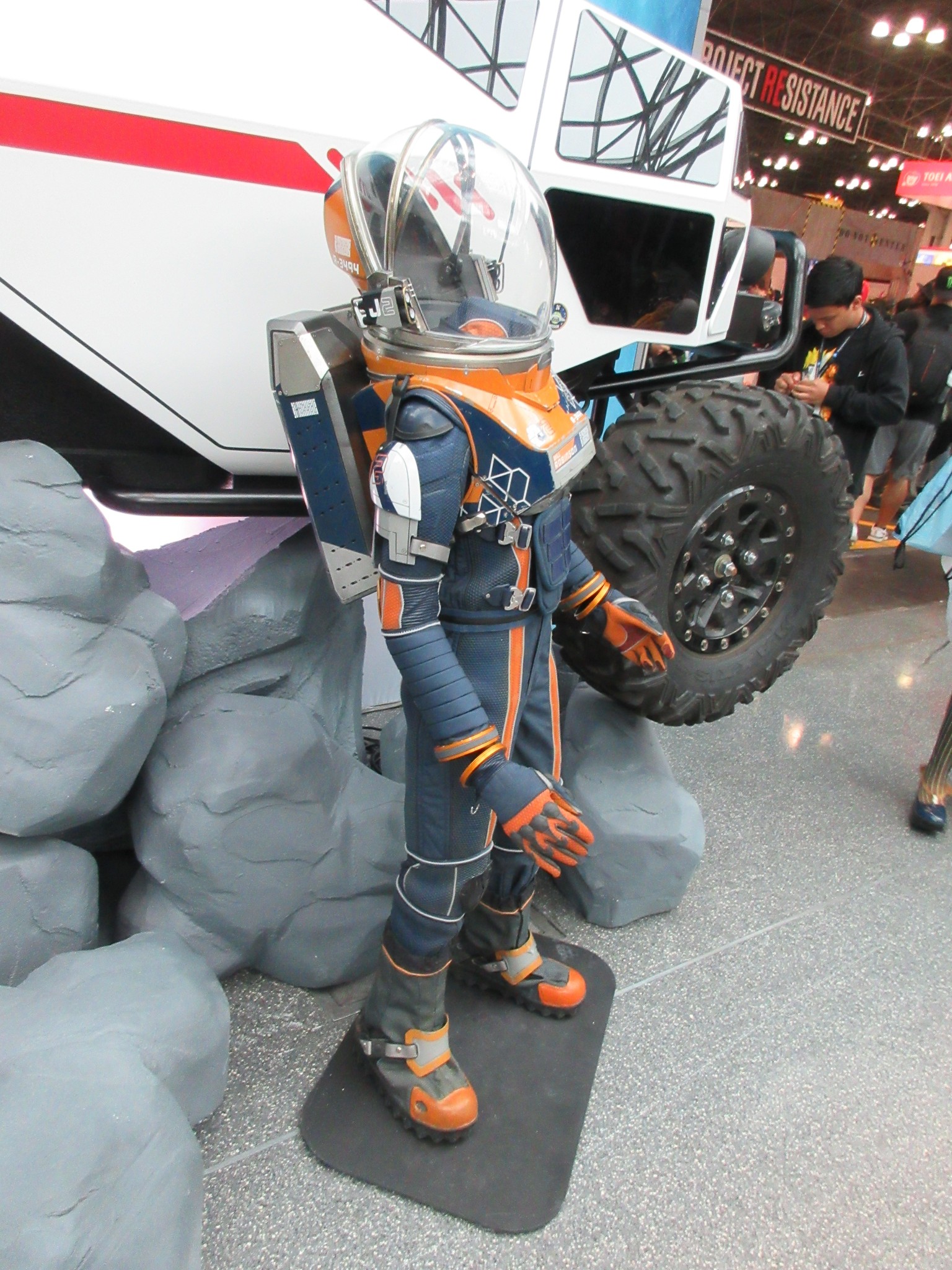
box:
[912,793,946,831]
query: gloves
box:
[574,583,675,672]
[459,743,594,878]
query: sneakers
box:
[866,525,888,541]
[849,525,859,542]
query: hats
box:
[935,266,952,290]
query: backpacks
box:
[905,307,952,404]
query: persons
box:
[910,695,952,835]
[322,119,675,1148]
[635,253,952,544]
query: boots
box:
[350,944,478,1145]
[450,890,587,1020]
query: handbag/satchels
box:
[891,441,952,572]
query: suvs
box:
[0,0,855,726]
